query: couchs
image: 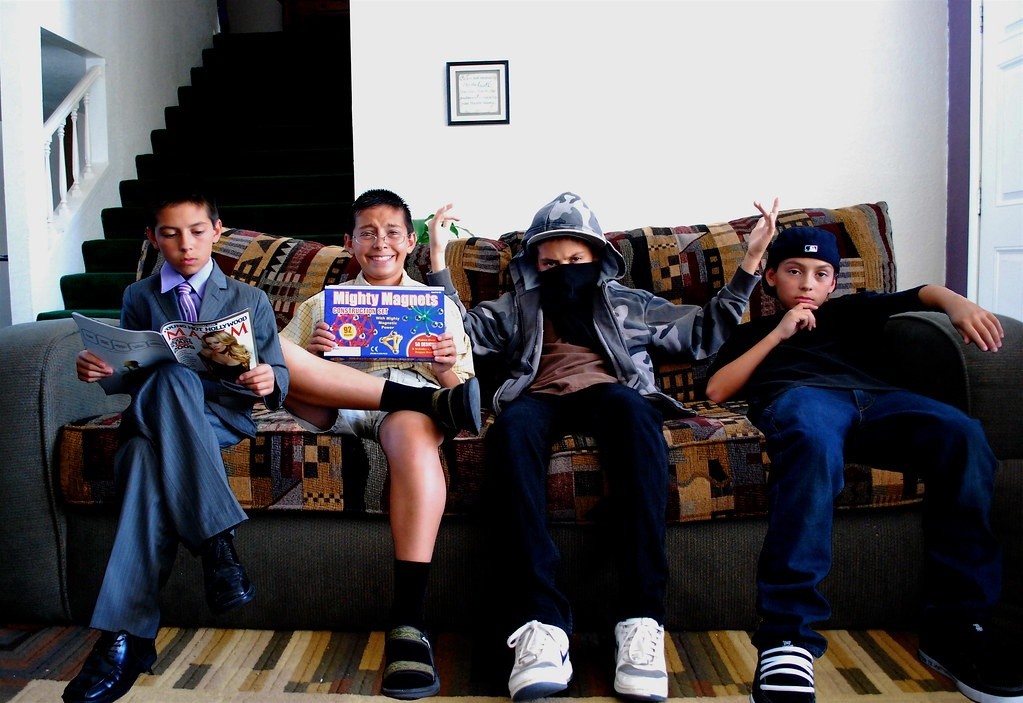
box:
[0,201,1023,630]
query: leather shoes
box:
[200,531,258,613]
[61,630,157,703]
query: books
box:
[71,308,263,398]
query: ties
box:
[177,282,199,322]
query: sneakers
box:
[507,619,573,703]
[613,616,669,702]
[916,623,1023,703]
[748,643,818,703]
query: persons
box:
[705,226,1023,703]
[196,331,251,386]
[427,193,781,703]
[59,186,291,703]
[276,189,482,699]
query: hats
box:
[759,225,841,297]
[526,229,607,247]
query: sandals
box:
[382,626,442,699]
[428,376,484,436]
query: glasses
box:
[351,230,412,246]
